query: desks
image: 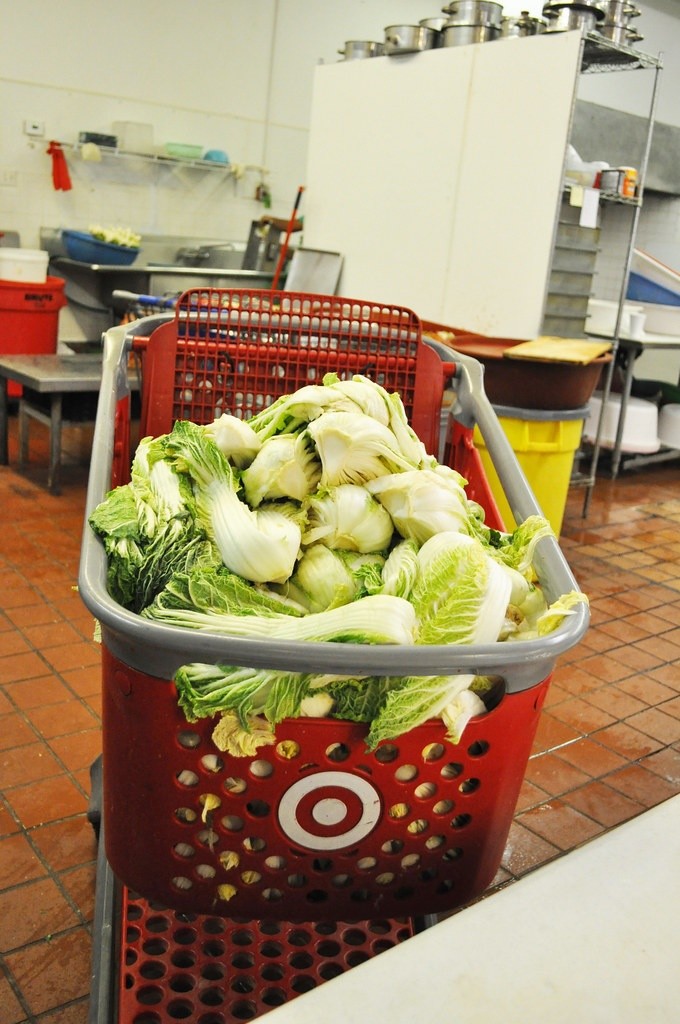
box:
[0,350,152,490]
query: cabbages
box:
[87,372,589,900]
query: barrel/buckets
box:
[0,275,66,395]
[474,403,591,582]
[0,246,50,286]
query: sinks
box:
[146,259,287,297]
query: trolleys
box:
[79,286,592,1023]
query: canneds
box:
[600,165,637,196]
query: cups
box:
[630,314,646,335]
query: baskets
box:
[62,230,143,265]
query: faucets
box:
[175,248,211,262]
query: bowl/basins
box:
[579,389,662,453]
[656,402,679,452]
[61,230,139,266]
[444,334,615,411]
[337,0,645,61]
